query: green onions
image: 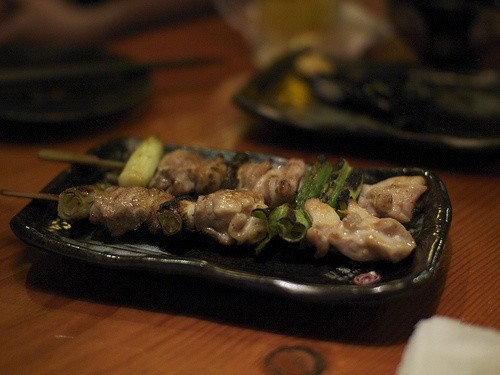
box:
[251,158,364,256]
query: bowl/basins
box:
[0,42,143,133]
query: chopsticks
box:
[4,53,215,80]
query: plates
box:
[232,42,498,161]
[12,131,451,309]
